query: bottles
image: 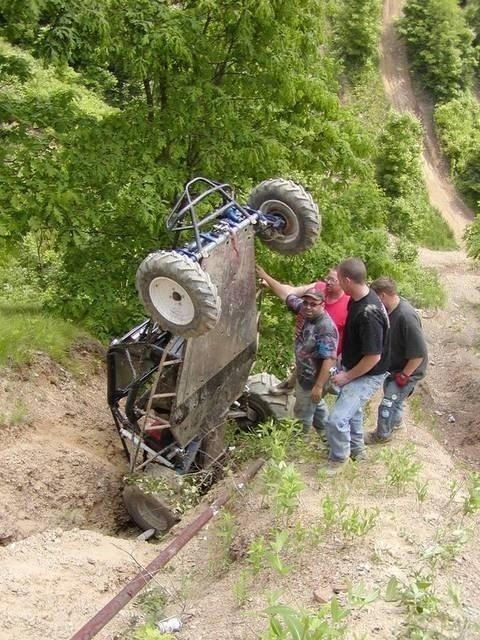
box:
[300,287,324,302]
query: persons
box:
[258,268,349,396]
[255,264,339,464]
[317,256,391,475]
[363,276,429,446]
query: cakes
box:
[328,366,338,376]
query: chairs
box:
[317,458,348,478]
[364,429,394,445]
